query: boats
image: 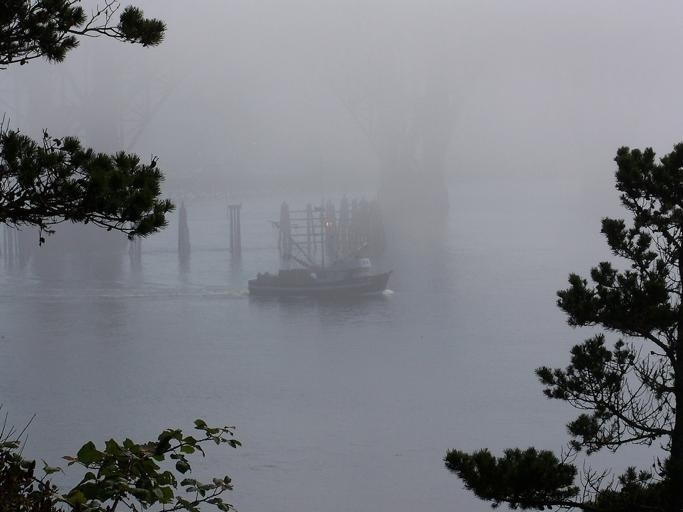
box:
[247,257,391,300]
